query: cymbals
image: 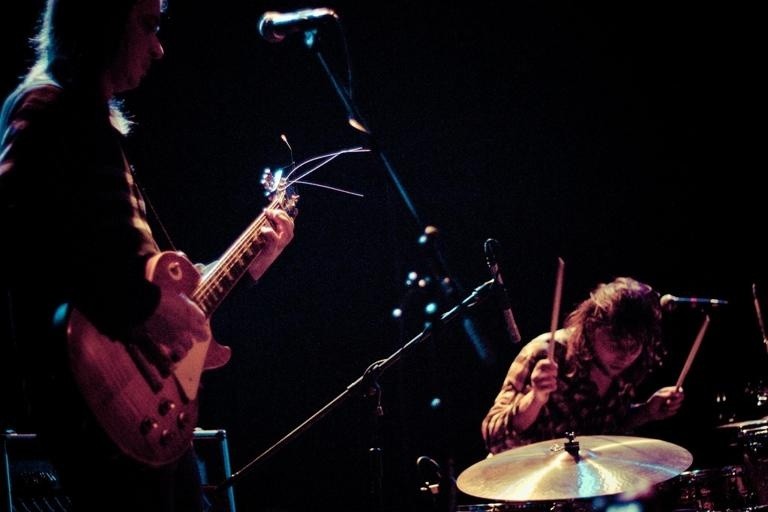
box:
[455,434,692,502]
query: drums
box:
[654,465,751,512]
[717,420,768,512]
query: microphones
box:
[483,236,523,344]
[257,7,338,44]
[659,293,727,311]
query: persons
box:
[1,1,297,512]
[480,277,685,458]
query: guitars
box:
[49,168,302,471]
